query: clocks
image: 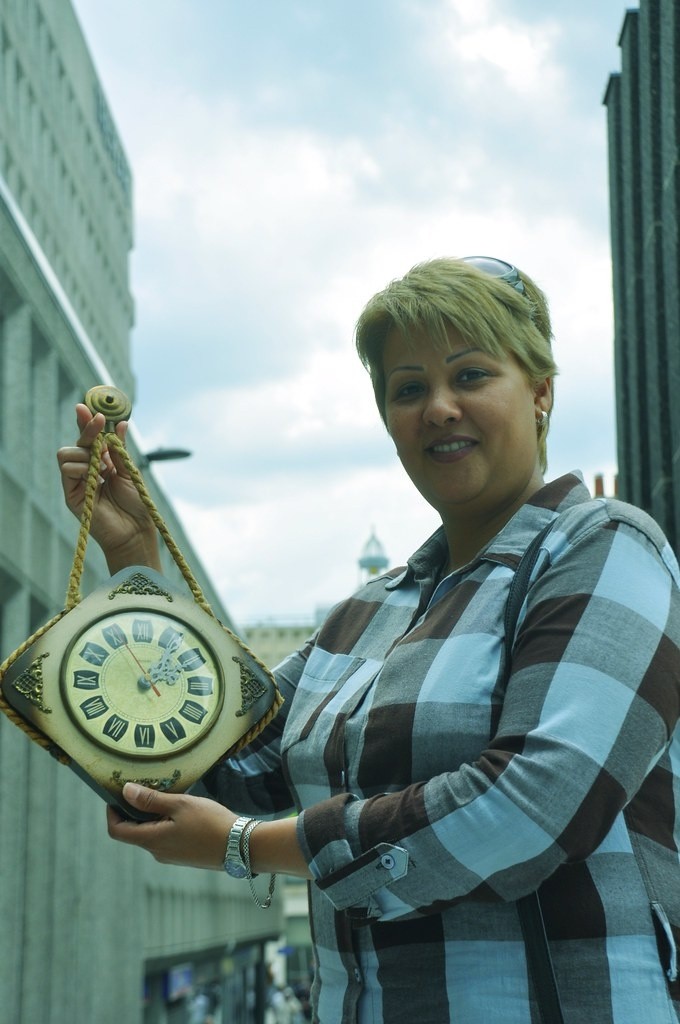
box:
[0,384,285,825]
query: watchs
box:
[224,815,261,882]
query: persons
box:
[104,252,680,1024]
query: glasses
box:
[460,255,542,338]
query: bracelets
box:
[242,819,277,909]
